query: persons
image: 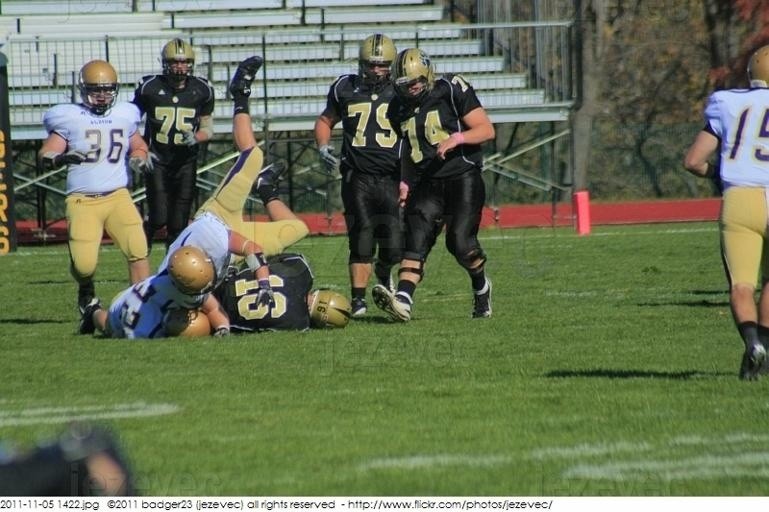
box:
[683,44,769,379]
[37,61,149,315]
[159,56,310,306]
[216,253,353,333]
[80,276,230,337]
[129,37,214,256]
[315,34,410,314]
[372,47,495,322]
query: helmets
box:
[309,288,352,329]
[162,38,195,83]
[80,60,119,115]
[747,44,769,88]
[166,308,211,337]
[358,33,397,84]
[168,246,215,295]
[389,48,436,106]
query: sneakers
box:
[80,296,103,334]
[738,342,767,380]
[78,277,95,315]
[350,258,491,322]
[228,57,263,96]
[253,159,289,194]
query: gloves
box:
[129,148,160,175]
[53,148,89,168]
[254,278,273,307]
[179,129,199,147]
[318,145,338,174]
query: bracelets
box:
[706,158,720,181]
[399,181,409,192]
[449,131,464,145]
